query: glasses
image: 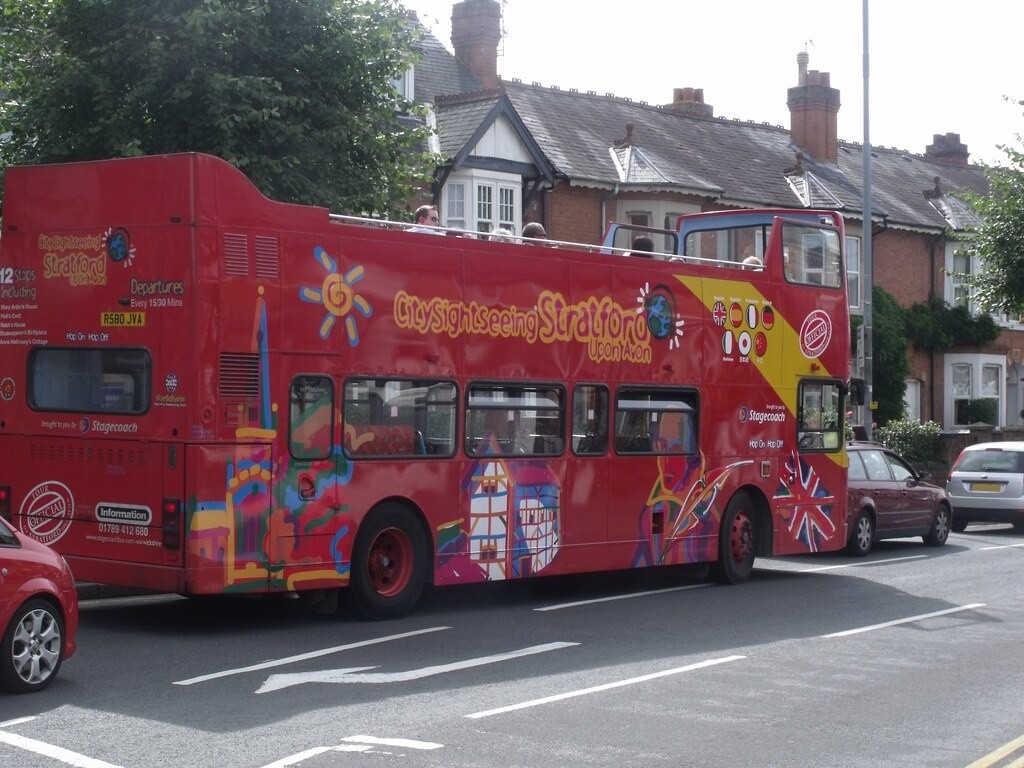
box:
[421,215,440,223]
[530,233,548,239]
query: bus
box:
[0,150,868,622]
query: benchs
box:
[345,424,426,455]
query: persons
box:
[403,205,473,239]
[742,256,762,272]
[872,419,876,430]
[521,222,547,247]
[852,426,869,444]
[489,228,514,243]
[630,236,653,258]
[846,408,853,418]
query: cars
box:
[845,438,953,557]
[0,515,80,696]
[946,441,1024,533]
